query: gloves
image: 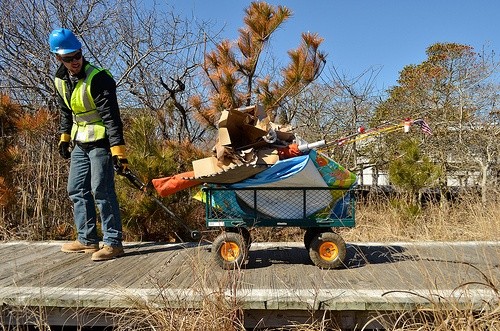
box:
[112,156,129,175]
[58,141,70,159]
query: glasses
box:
[61,52,82,62]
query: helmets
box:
[49,27,81,54]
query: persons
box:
[49,28,129,261]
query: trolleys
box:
[114,164,358,271]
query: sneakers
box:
[62,239,99,254]
[92,245,125,262]
